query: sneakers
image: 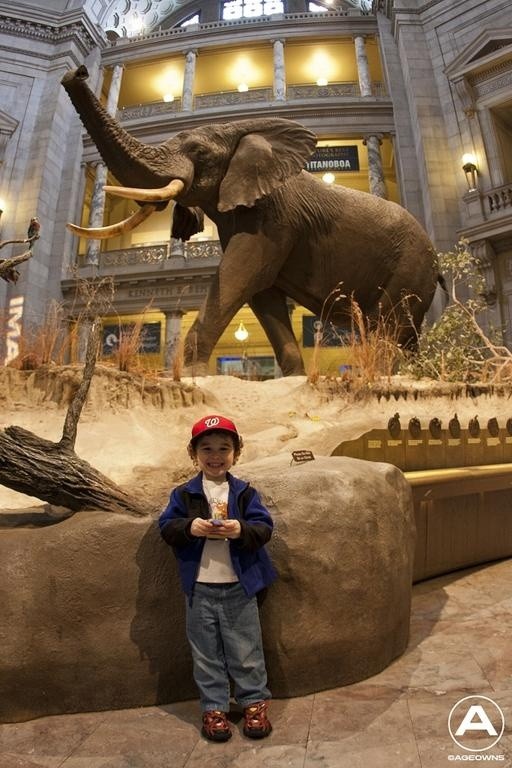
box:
[244,703,272,738]
[201,710,232,741]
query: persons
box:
[156,414,281,741]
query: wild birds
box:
[28,216,40,251]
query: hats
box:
[190,414,239,441]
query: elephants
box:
[60,66,450,378]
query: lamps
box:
[462,153,478,192]
[234,319,248,341]
